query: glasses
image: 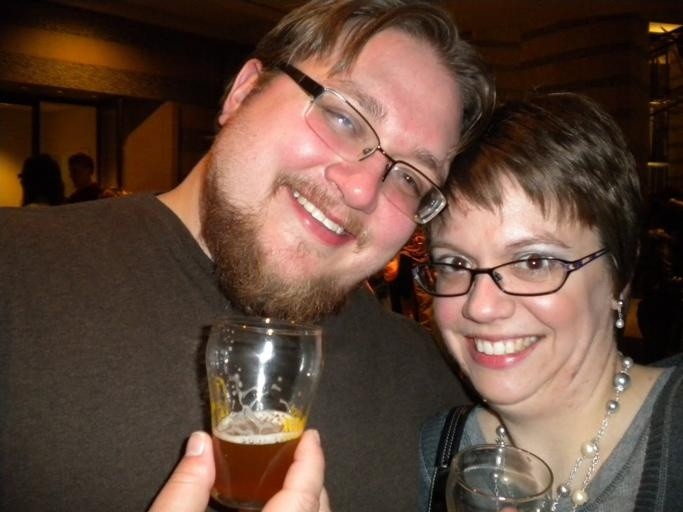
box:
[405,244,616,300]
[264,55,450,228]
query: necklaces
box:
[493,355,634,512]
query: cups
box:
[205,316,324,508]
[446,444,555,512]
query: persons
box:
[410,93,683,512]
[65,152,105,203]
[19,153,65,209]
[0,0,500,512]
